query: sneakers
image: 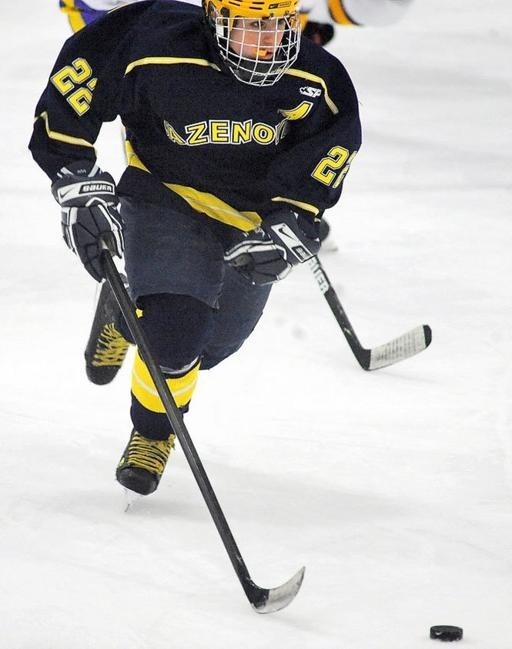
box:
[115,425,178,496]
[84,273,130,387]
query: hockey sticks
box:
[101,249,305,613]
[308,257,431,371]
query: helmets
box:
[200,0,303,34]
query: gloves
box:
[220,205,323,287]
[52,158,127,282]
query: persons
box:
[60,0,413,36]
[27,0,362,494]
